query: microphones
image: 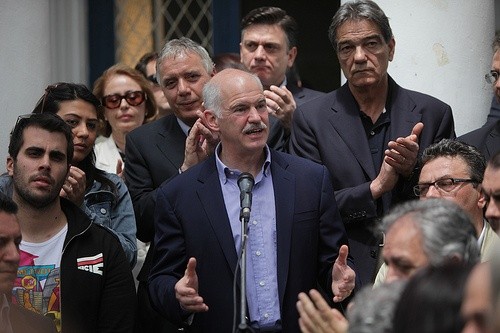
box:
[238,172,255,223]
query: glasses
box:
[102,91,146,110]
[485,70,500,85]
[413,177,477,196]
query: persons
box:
[296,138,500,333]
[93,37,249,333]
[456,31,500,162]
[290,0,457,284]
[0,114,137,332]
[147,68,359,333]
[0,193,58,333]
[240,7,327,154]
[0,82,138,270]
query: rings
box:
[276,106,280,111]
